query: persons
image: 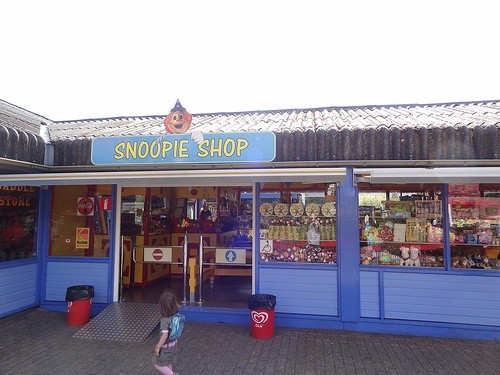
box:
[151,288,185,375]
[200,205,212,219]
[306,214,320,253]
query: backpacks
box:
[167,315,186,341]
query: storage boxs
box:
[362,200,442,243]
[448,183,500,220]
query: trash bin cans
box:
[65,285,94,326]
[248,294,277,339]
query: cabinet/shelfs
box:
[357,215,445,268]
[449,184,499,270]
[260,213,336,264]
